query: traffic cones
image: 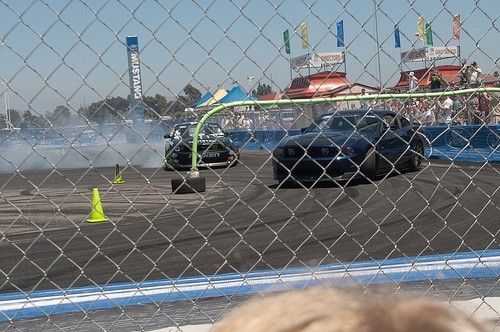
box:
[112,164,125,184]
[87,188,109,222]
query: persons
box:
[407,72,418,90]
[429,68,442,90]
[462,62,483,85]
[220,81,500,127]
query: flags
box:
[452,14,460,40]
[336,20,344,47]
[393,24,400,48]
[300,20,308,49]
[417,16,425,42]
[283,29,290,55]
[426,23,433,45]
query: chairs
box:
[180,128,189,135]
[205,127,213,135]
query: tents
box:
[189,86,258,112]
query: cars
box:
[162,122,240,171]
[273,110,423,188]
